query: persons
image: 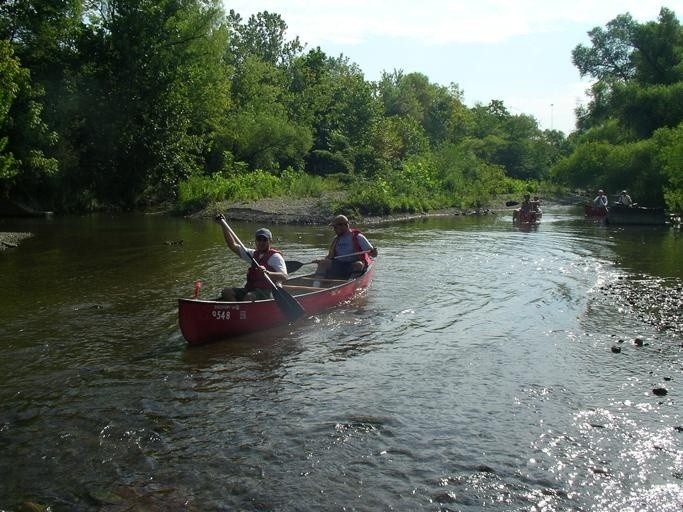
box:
[521,193,541,213]
[617,189,632,206]
[214,213,288,301]
[593,189,608,207]
[311,214,378,288]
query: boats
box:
[176,256,375,345]
[511,205,665,231]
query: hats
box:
[621,190,627,194]
[255,228,272,240]
[328,215,349,227]
[598,190,603,193]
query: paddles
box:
[219,220,306,324]
[506,202,523,207]
[285,247,374,274]
